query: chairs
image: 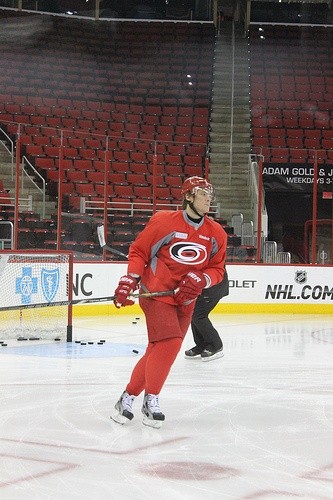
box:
[0,8,333,270]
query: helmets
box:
[181,177,212,195]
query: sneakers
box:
[184,346,202,359]
[140,393,165,428]
[202,345,224,360]
[110,390,135,425]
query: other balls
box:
[133,350,138,354]
[54,338,60,341]
[136,318,140,319]
[88,342,93,344]
[75,340,80,343]
[98,342,103,344]
[132,322,137,324]
[0,337,40,347]
[81,343,86,345]
[100,340,105,342]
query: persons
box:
[0,213,12,244]
[107,175,228,430]
[183,263,230,362]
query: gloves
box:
[114,274,137,308]
[173,272,207,306]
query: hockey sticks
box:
[81,290,173,304]
[97,225,129,259]
